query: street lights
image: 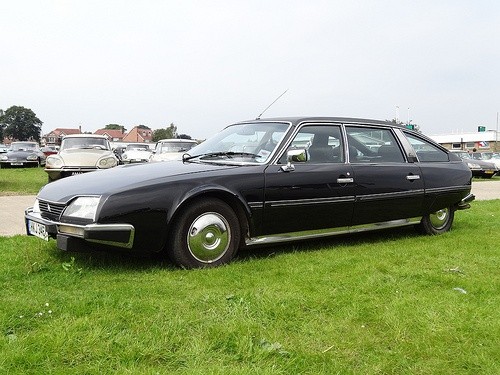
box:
[408,108,411,126]
[395,106,399,124]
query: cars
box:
[0,140,47,170]
[0,139,156,156]
[226,132,500,179]
[23,116,477,269]
[119,143,154,165]
[148,138,199,165]
[43,132,120,183]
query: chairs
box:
[263,143,286,164]
[309,144,333,162]
[378,145,402,162]
[333,145,358,162]
